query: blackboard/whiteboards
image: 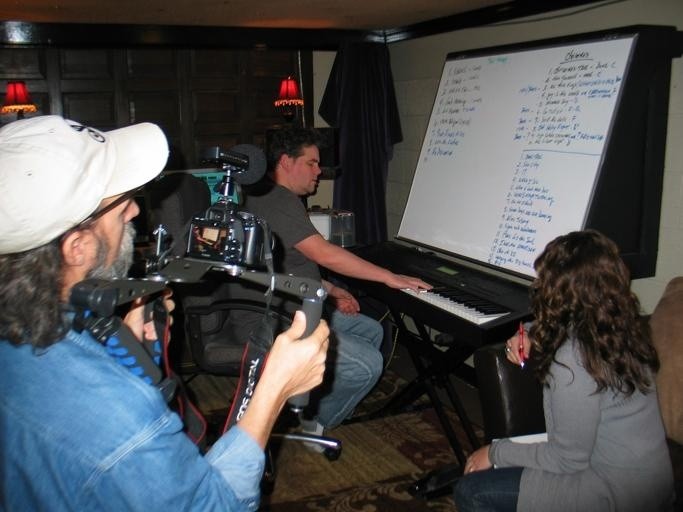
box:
[393,33,639,282]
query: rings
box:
[505,347,511,353]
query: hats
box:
[0,115,170,257]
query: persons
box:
[453,229,678,512]
[241,126,434,430]
[0,115,330,512]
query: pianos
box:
[311,240,538,347]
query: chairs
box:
[474,277,683,512]
[146,171,342,496]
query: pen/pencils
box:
[519,321,525,369]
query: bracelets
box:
[514,322,532,336]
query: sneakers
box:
[295,410,326,454]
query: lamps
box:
[0,79,39,121]
[273,76,304,115]
